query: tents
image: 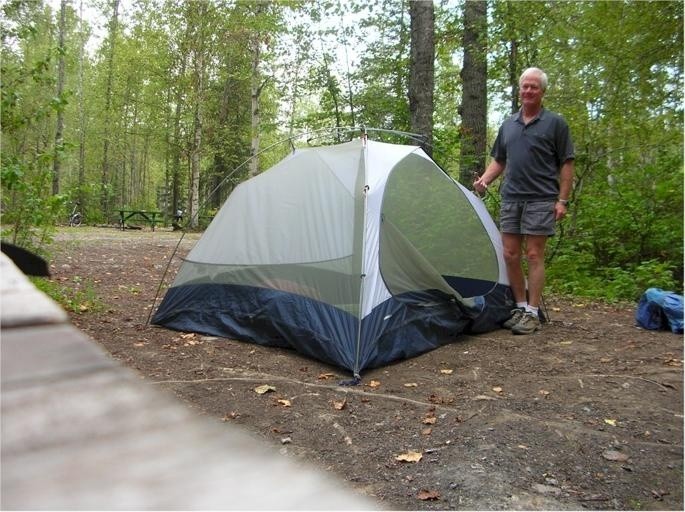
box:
[146,127,551,384]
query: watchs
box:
[558,198,570,208]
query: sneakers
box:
[503,306,526,330]
[511,311,543,334]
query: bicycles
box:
[68,200,83,228]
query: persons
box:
[472,66,576,333]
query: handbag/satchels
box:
[634,288,684,334]
